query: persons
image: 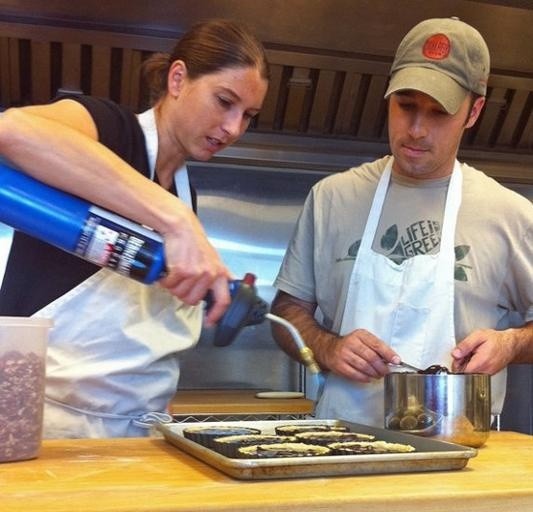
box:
[270,15,533,430]
[0,18,272,440]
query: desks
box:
[0,386,533,511]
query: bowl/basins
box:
[384,371,492,446]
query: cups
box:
[0,318,53,460]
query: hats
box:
[383,16,491,116]
